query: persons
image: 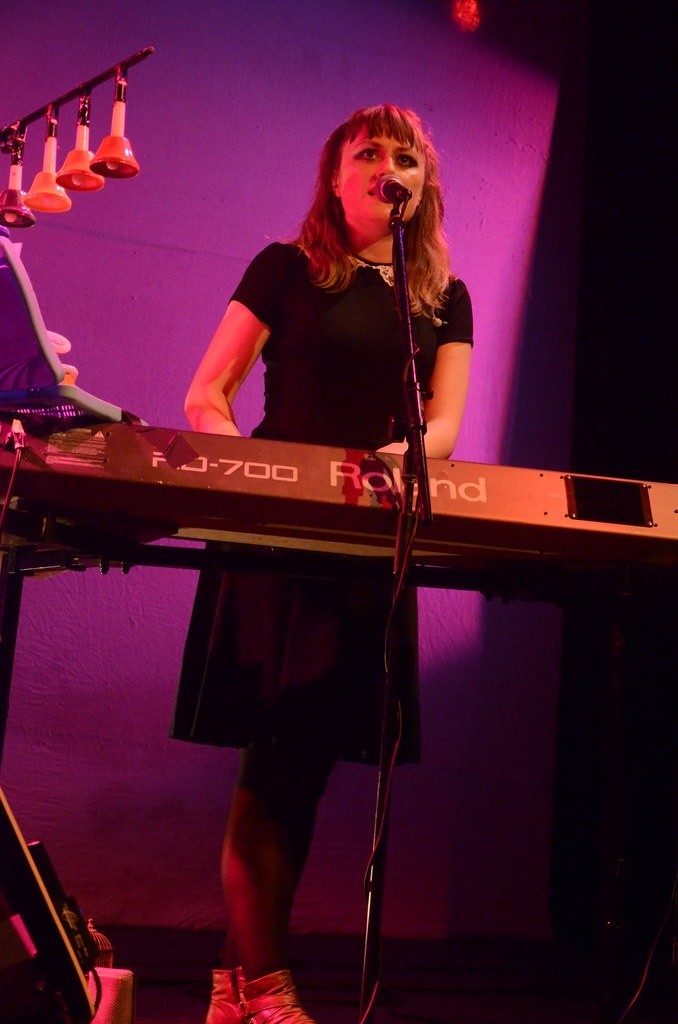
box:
[166,103,474,1024]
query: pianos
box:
[0,392,678,616]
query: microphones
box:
[375,175,412,204]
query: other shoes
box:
[238,969,314,1024]
[206,970,245,1024]
